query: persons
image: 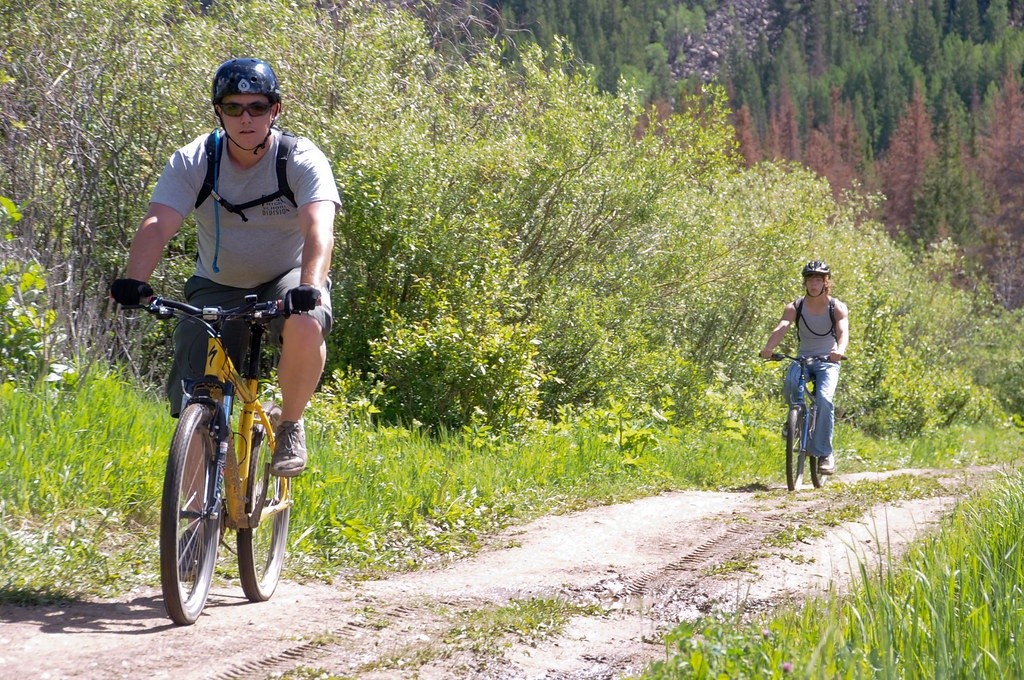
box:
[113,60,338,584]
[760,261,850,474]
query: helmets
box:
[211,57,280,105]
[802,261,830,277]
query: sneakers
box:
[178,529,202,582]
[782,420,798,441]
[818,453,835,475]
[268,417,308,478]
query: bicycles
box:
[111,284,293,623]
[761,345,847,492]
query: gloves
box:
[284,284,321,320]
[110,278,153,306]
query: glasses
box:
[217,102,274,117]
